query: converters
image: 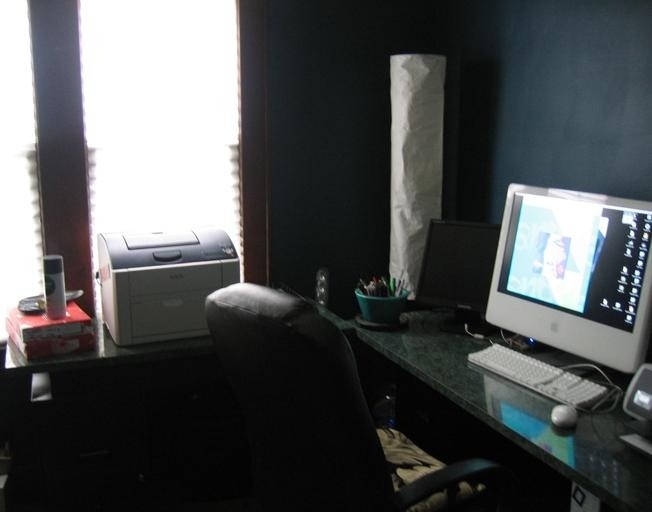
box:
[525,336,546,354]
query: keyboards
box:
[468,343,609,410]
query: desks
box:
[347,308,652,512]
[5,293,355,512]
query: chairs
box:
[205,283,517,511]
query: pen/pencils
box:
[357,269,406,297]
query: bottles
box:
[44,255,66,318]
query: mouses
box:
[552,404,578,428]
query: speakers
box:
[620,363,652,421]
[314,266,334,311]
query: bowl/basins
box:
[355,286,408,324]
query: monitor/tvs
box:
[485,183,652,384]
[415,218,501,335]
[485,373,631,503]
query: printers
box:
[98,230,239,347]
